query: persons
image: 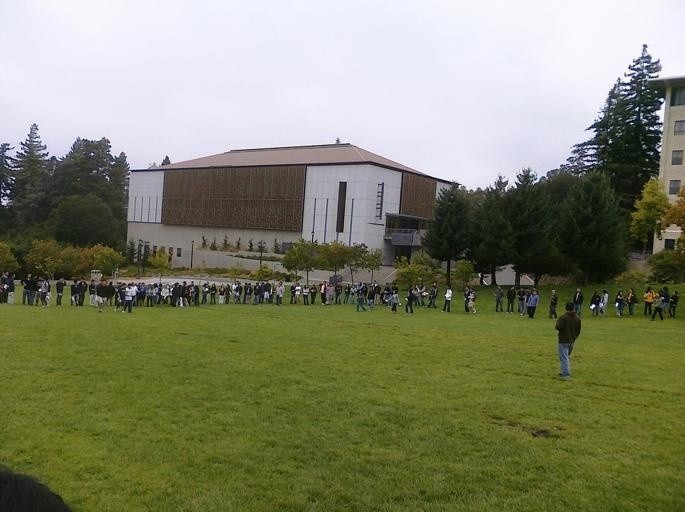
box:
[467,288,477,314]
[441,285,453,312]
[0,271,51,308]
[615,291,627,318]
[56,277,66,307]
[644,286,679,321]
[600,289,608,317]
[627,288,637,317]
[89,279,200,312]
[201,281,286,307]
[549,290,558,318]
[573,288,584,316]
[555,302,582,381]
[464,286,470,312]
[464,280,477,291]
[404,280,437,313]
[590,291,601,317]
[71,277,88,306]
[290,280,399,313]
[493,284,539,318]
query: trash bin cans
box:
[91,270,101,282]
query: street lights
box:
[137,239,143,272]
[311,231,314,243]
[190,241,194,268]
[258,241,266,269]
